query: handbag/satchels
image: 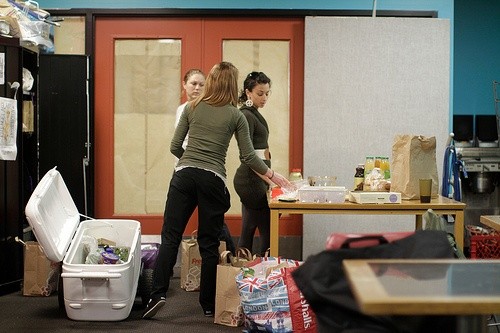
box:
[291,230,488,333]
[391,134,439,200]
[214,248,252,327]
[235,248,318,333]
[180,230,227,292]
[18,239,61,297]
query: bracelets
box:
[270,170,274,179]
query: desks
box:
[264,193,468,257]
[480,214,500,232]
[342,259,500,317]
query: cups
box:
[419,179,432,203]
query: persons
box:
[136,62,292,319]
[175,69,236,263]
[233,71,272,257]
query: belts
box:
[254,148,272,161]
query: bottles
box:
[355,155,391,191]
[290,168,301,180]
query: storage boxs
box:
[25,165,142,321]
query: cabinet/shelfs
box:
[0,31,92,297]
